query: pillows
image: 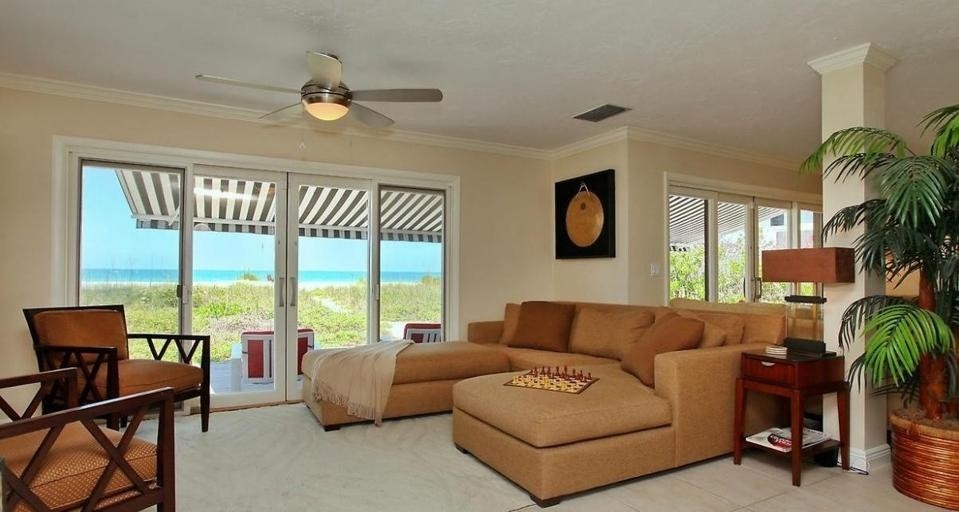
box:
[500,301,520,343]
[510,301,576,350]
[620,308,704,386]
[656,306,727,348]
[698,312,744,345]
[570,306,659,358]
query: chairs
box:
[240,329,315,390]
[23,301,211,432]
[0,368,175,512]
[403,324,441,342]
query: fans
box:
[194,50,443,128]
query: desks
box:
[730,351,850,486]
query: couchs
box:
[459,298,788,510]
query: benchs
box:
[302,341,511,434]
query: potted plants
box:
[797,105,959,512]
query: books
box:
[745,426,831,454]
